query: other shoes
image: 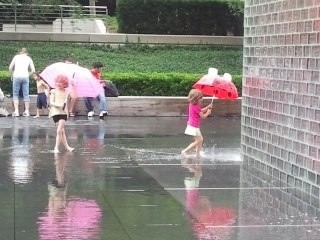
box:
[87,110,94,117]
[12,112,19,116]
[99,111,108,118]
[23,111,30,116]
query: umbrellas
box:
[39,196,102,240]
[39,61,103,110]
[192,67,238,104]
[192,207,237,240]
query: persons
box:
[9,48,36,116]
[33,71,51,118]
[46,153,74,221]
[181,89,212,157]
[81,62,108,117]
[8,116,35,187]
[82,119,106,155]
[182,158,211,220]
[48,75,77,153]
